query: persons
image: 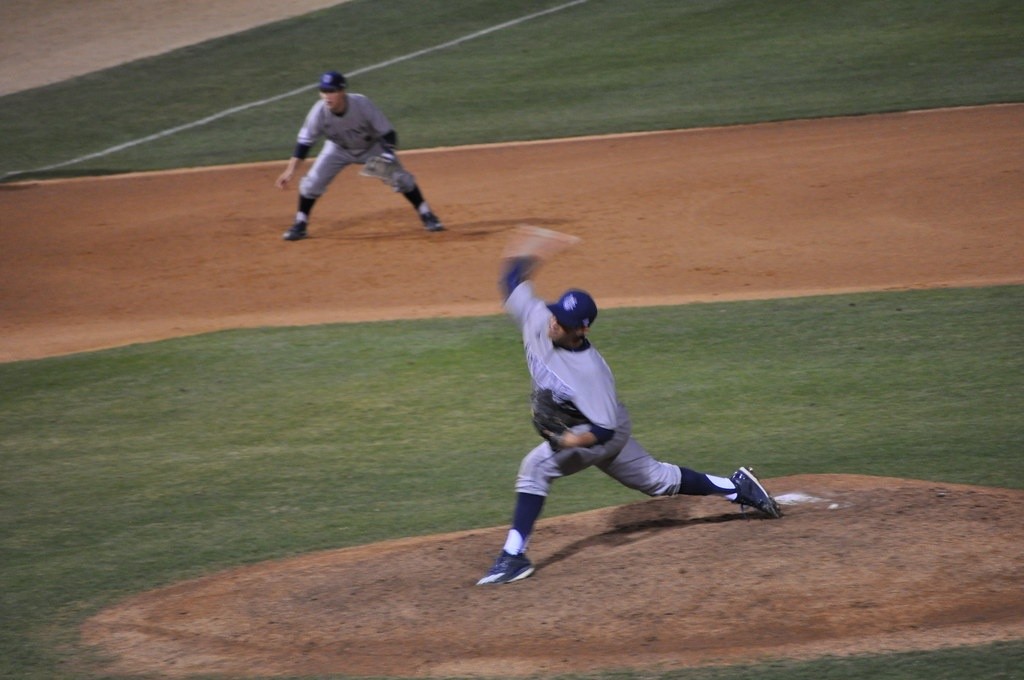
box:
[474,224,783,584]
[277,70,444,240]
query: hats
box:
[546,288,597,328]
[316,70,348,92]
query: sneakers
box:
[282,222,307,239]
[474,548,535,585]
[728,465,781,519]
[420,211,442,231]
